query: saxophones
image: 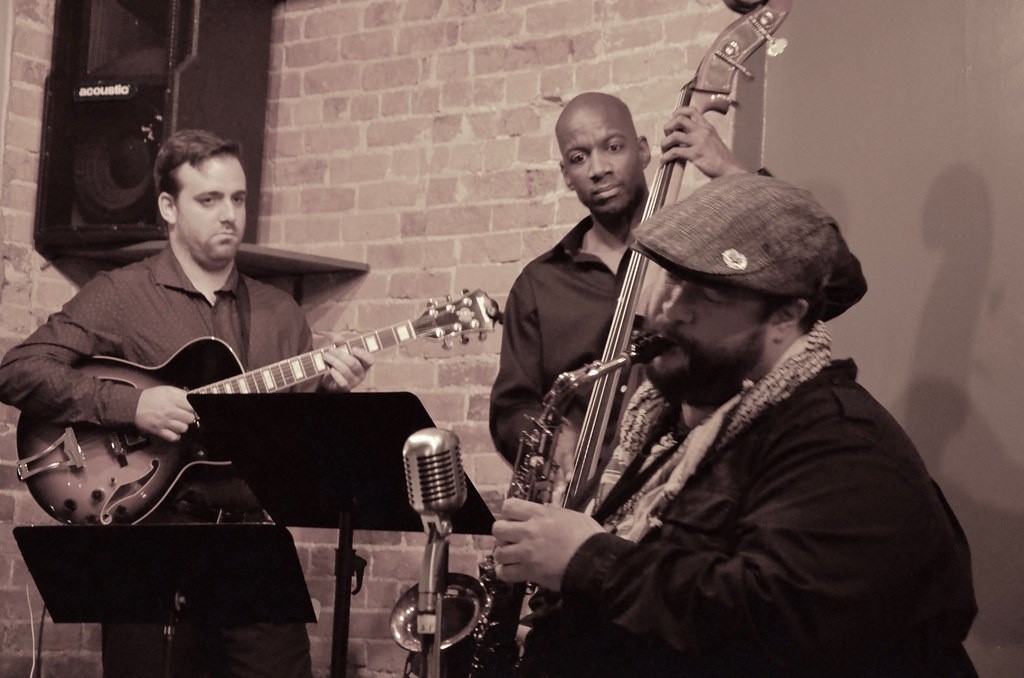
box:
[388,331,661,677]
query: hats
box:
[629,172,870,328]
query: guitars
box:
[16,287,504,526]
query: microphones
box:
[404,426,467,636]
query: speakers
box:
[32,2,269,261]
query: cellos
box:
[562,1,791,510]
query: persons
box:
[489,163,990,678]
[486,92,775,475]
[0,126,378,676]
